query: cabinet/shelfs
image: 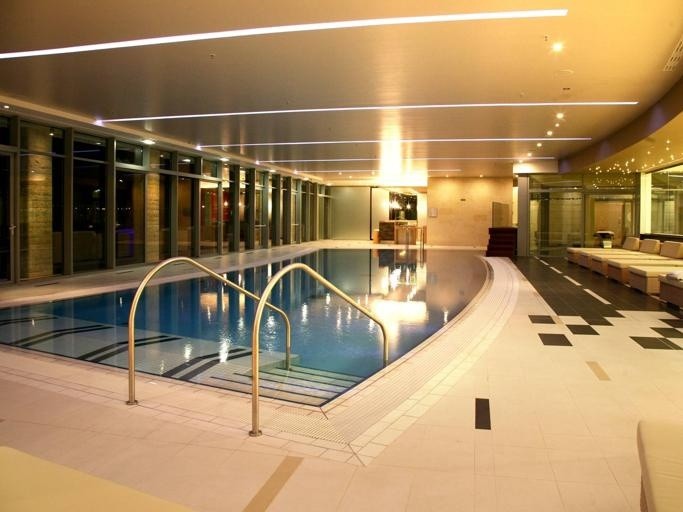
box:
[378,217,409,243]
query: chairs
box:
[634,409,682,512]
[563,234,682,307]
